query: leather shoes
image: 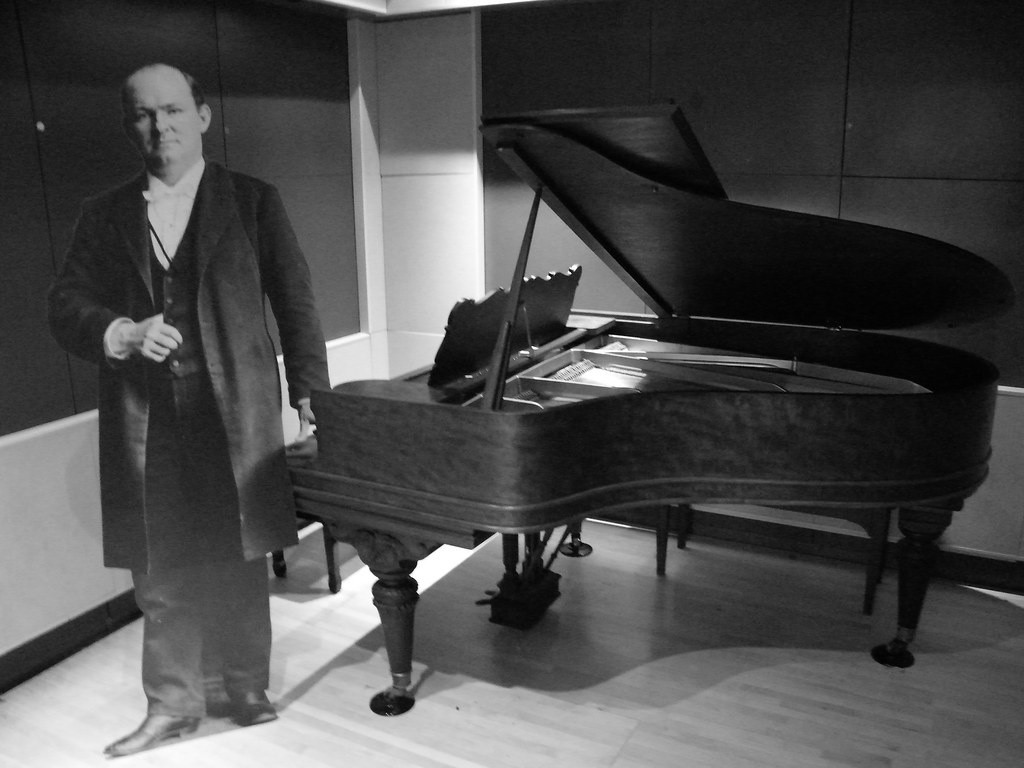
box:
[104,714,200,756]
[206,687,277,727]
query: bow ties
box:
[142,189,194,212]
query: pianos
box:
[284,100,1017,716]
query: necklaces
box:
[147,219,179,276]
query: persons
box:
[48,62,332,756]
